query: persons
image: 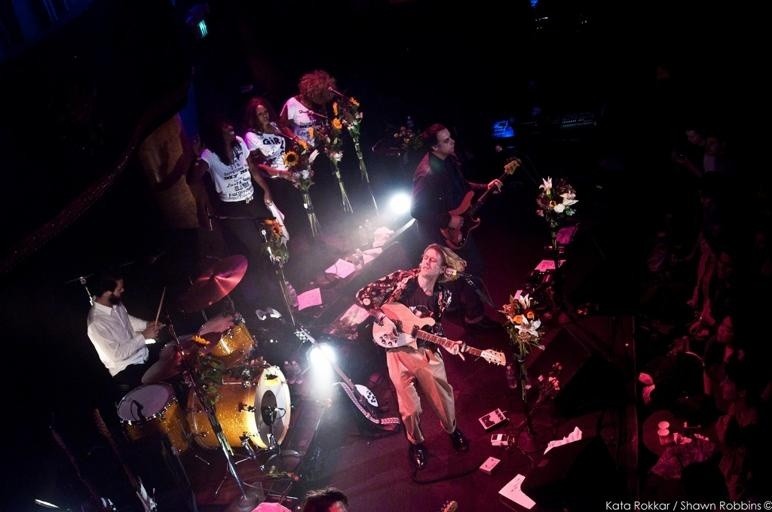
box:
[301,487,348,512]
[628,117,772,512]
[354,243,467,470]
[410,125,504,285]
[88,274,167,387]
[138,68,348,272]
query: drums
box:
[117,382,191,459]
[143,362,182,383]
[187,363,293,452]
[162,333,196,370]
[198,311,256,371]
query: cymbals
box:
[177,254,248,311]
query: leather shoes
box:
[413,444,427,471]
[450,427,470,453]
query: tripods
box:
[165,315,272,496]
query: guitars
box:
[373,301,507,371]
[443,158,522,248]
[255,304,399,436]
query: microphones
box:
[132,398,145,411]
[308,108,329,120]
[327,87,348,101]
[205,203,216,233]
[446,267,481,283]
[274,129,293,141]
